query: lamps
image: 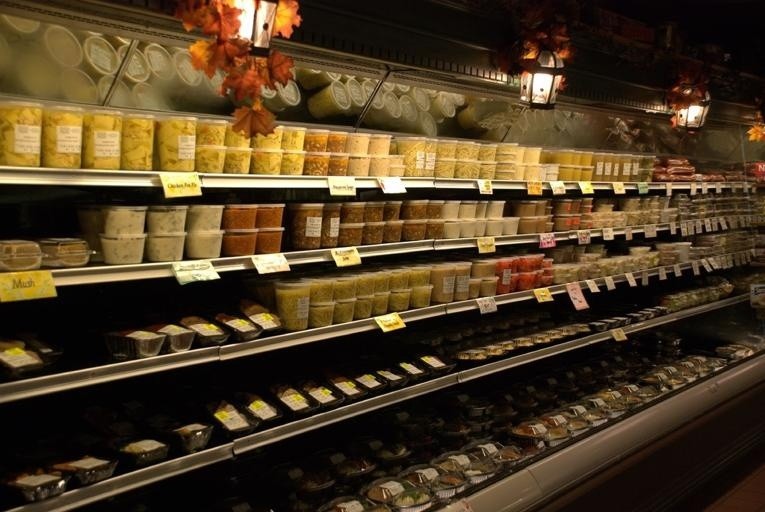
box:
[232,0,279,59]
[661,67,713,133]
[488,11,579,112]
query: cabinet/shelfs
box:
[0,164,765,510]
